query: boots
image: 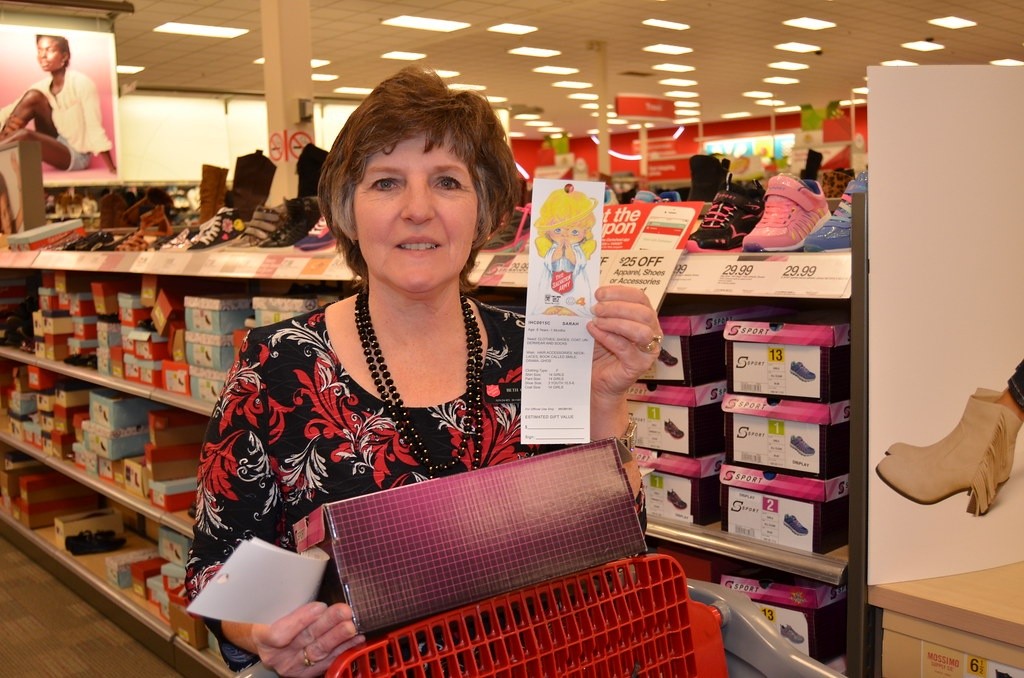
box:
[295,142,327,199]
[231,149,277,213]
[685,154,732,200]
[191,164,228,222]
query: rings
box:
[303,649,314,666]
[639,336,661,353]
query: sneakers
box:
[686,175,765,254]
[803,177,867,251]
[294,212,336,251]
[781,625,804,644]
[740,174,831,253]
[664,419,684,439]
[480,204,530,253]
[229,207,283,248]
[660,347,678,366]
[790,360,815,383]
[790,434,814,457]
[784,514,809,538]
[667,489,686,509]
[261,199,321,247]
[184,207,244,251]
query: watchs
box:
[619,414,639,451]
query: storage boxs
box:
[0,272,850,665]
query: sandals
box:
[118,205,173,252]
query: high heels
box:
[875,387,1022,518]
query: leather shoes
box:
[65,230,113,251]
[65,529,127,556]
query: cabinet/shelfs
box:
[0,56,1024,678]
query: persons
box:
[184,67,663,678]
[879,353,1024,517]
[0,34,116,171]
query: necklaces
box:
[357,286,483,478]
